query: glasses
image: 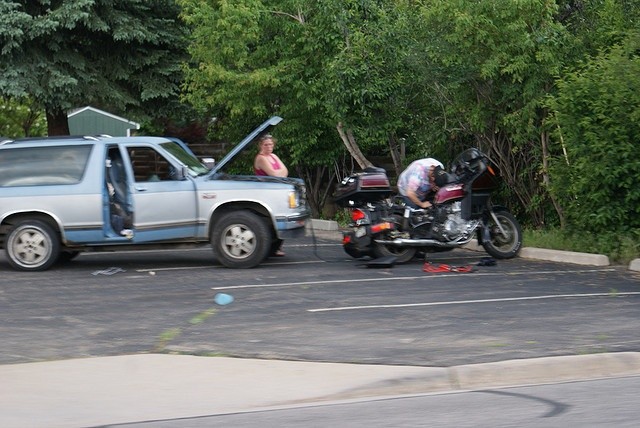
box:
[262,135,272,140]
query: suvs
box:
[0,116,310,272]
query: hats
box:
[434,165,448,187]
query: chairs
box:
[108,159,139,206]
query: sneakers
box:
[275,250,285,256]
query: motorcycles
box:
[333,148,523,264]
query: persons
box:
[255,134,289,258]
[397,155,450,268]
